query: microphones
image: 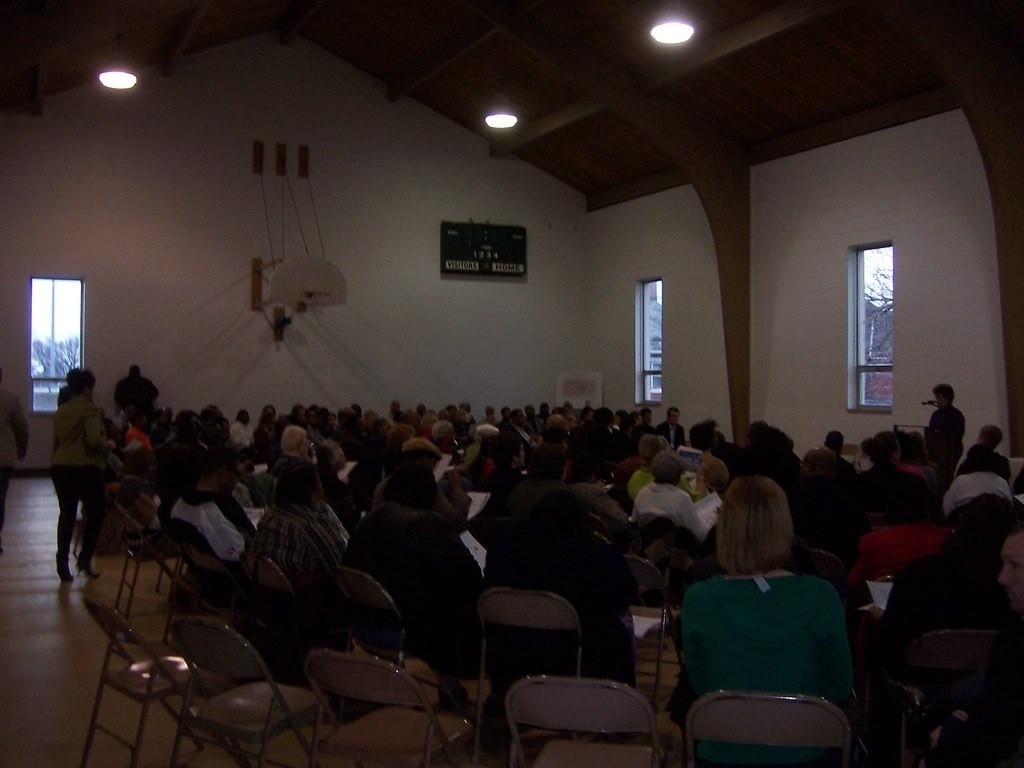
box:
[922,400,936,405]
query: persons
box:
[929,383,966,494]
[114,365,159,410]
[0,369,29,554]
[104,401,1024,768]
[681,474,855,767]
[52,368,117,583]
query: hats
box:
[651,450,687,481]
[402,436,442,462]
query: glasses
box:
[799,459,807,467]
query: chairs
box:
[81,497,1002,768]
[837,443,861,469]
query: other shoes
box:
[443,687,467,711]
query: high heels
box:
[77,559,100,579]
[57,565,73,583]
